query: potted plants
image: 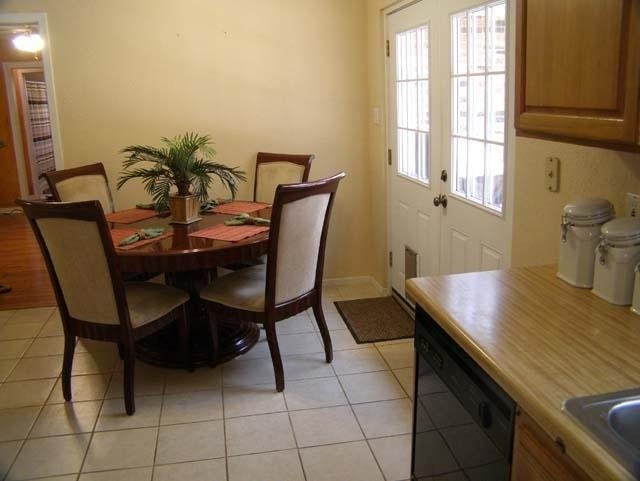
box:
[116,130,248,227]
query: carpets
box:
[333,296,415,345]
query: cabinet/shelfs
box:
[514,1,640,154]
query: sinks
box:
[564,385,640,479]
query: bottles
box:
[558,196,640,307]
